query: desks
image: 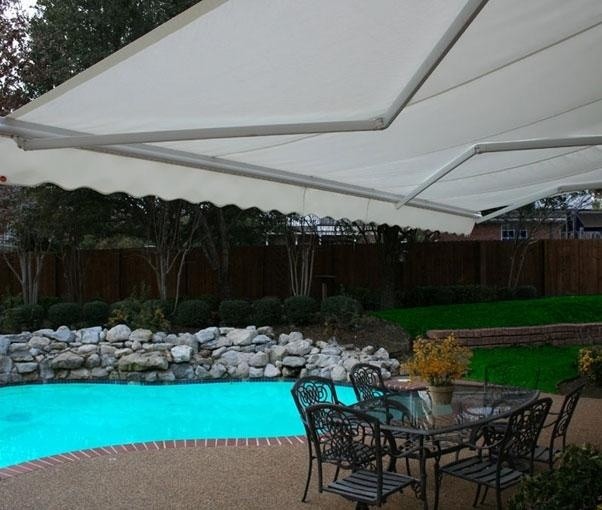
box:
[324,383,541,509]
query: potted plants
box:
[398,330,472,403]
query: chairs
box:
[292,364,589,510]
